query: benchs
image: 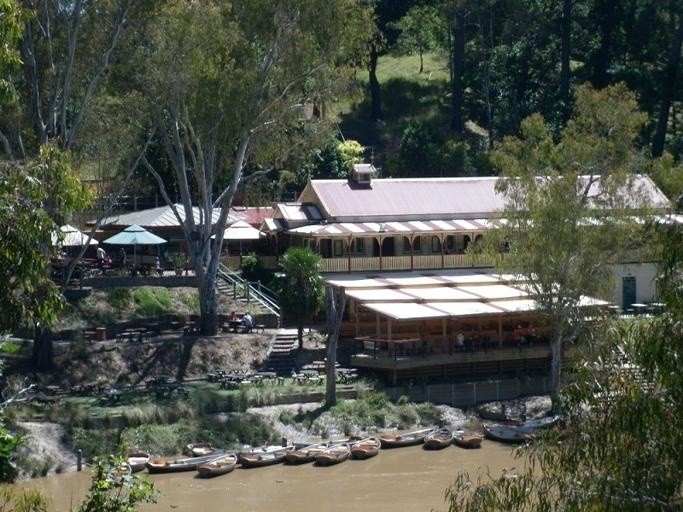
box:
[602,296,665,319]
[26,319,551,408]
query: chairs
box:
[107,256,164,276]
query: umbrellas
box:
[46,221,98,259]
[207,219,267,256]
[101,222,170,270]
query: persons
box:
[96,245,106,266]
[225,311,238,331]
[118,247,127,272]
[241,309,253,333]
[152,255,161,271]
